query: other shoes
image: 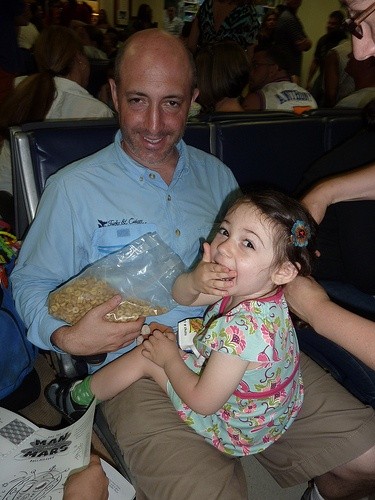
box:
[44,380,97,425]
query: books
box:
[1,396,136,500]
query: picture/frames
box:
[113,0,132,30]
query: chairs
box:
[9,108,375,499]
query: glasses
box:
[344,2,375,39]
[250,60,276,72]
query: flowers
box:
[290,220,311,247]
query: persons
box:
[43,188,319,456]
[281,167,375,408]
[0,0,375,231]
[9,29,375,500]
[63,454,109,500]
